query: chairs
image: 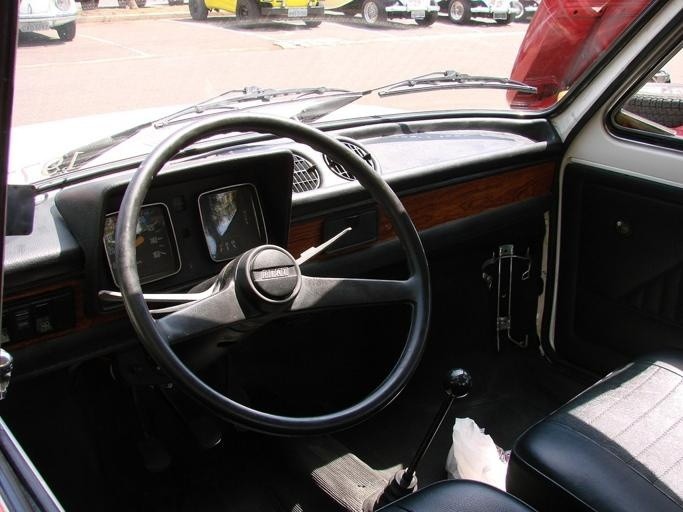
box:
[504,353,683,508]
[361,478,534,507]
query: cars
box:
[19,0,82,43]
[437,1,521,27]
[187,0,326,28]
[326,0,441,31]
